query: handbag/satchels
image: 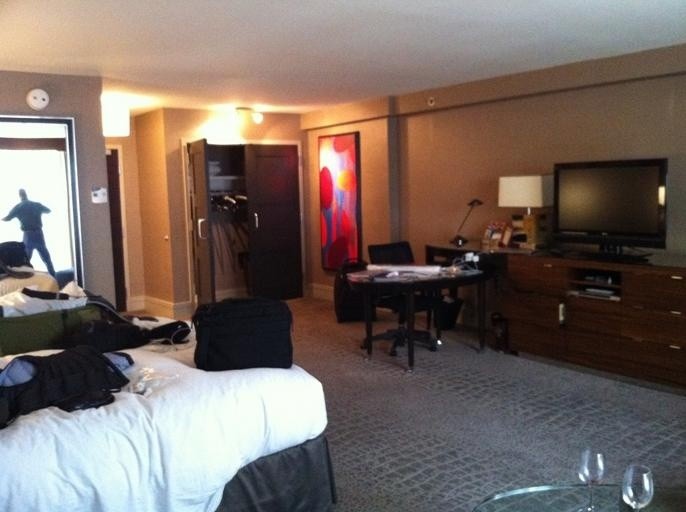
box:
[191,294,297,372]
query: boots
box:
[489,311,517,355]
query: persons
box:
[1,187,57,278]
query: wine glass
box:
[576,450,608,512]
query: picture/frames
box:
[319,130,361,271]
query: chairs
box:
[333,240,509,369]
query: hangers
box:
[209,188,246,211]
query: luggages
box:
[331,257,378,324]
[0,289,133,359]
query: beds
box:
[0,317,338,512]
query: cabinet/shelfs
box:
[504,247,686,387]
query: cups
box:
[621,464,655,512]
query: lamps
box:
[497,172,553,252]
[449,198,483,246]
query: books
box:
[347,272,372,278]
[455,269,480,278]
[582,287,615,295]
[576,292,621,303]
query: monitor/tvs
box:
[552,157,668,264]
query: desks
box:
[470,484,686,512]
